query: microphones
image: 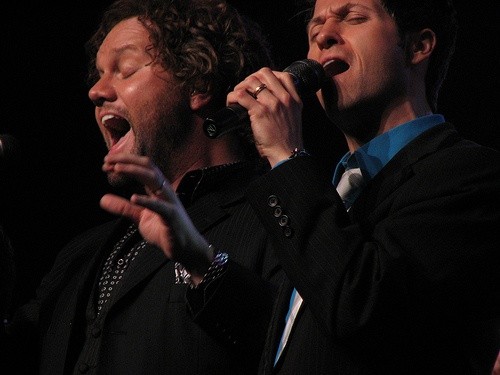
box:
[203,59,325,139]
[0,134,16,158]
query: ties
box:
[273,167,363,368]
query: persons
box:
[225,1,500,372]
[0,0,282,372]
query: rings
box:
[152,179,170,194]
[250,83,268,98]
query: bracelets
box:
[172,243,231,294]
[289,146,316,162]
